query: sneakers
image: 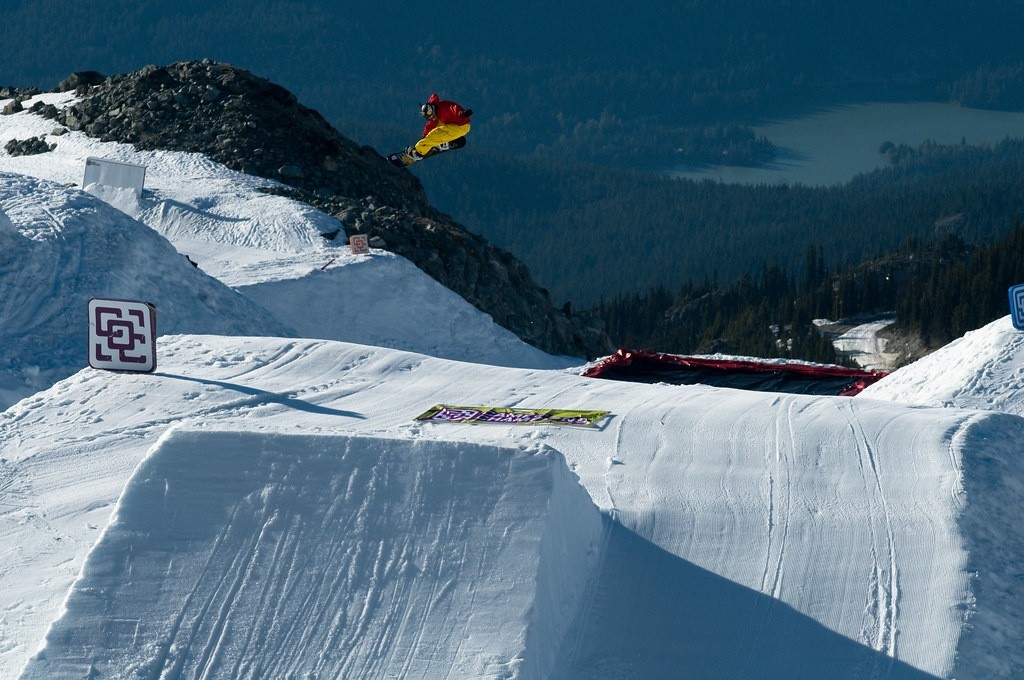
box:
[404,146,422,162]
[438,143,449,151]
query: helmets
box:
[418,103,435,121]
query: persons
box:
[405,94,473,160]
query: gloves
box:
[461,108,473,118]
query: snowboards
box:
[385,136,467,168]
